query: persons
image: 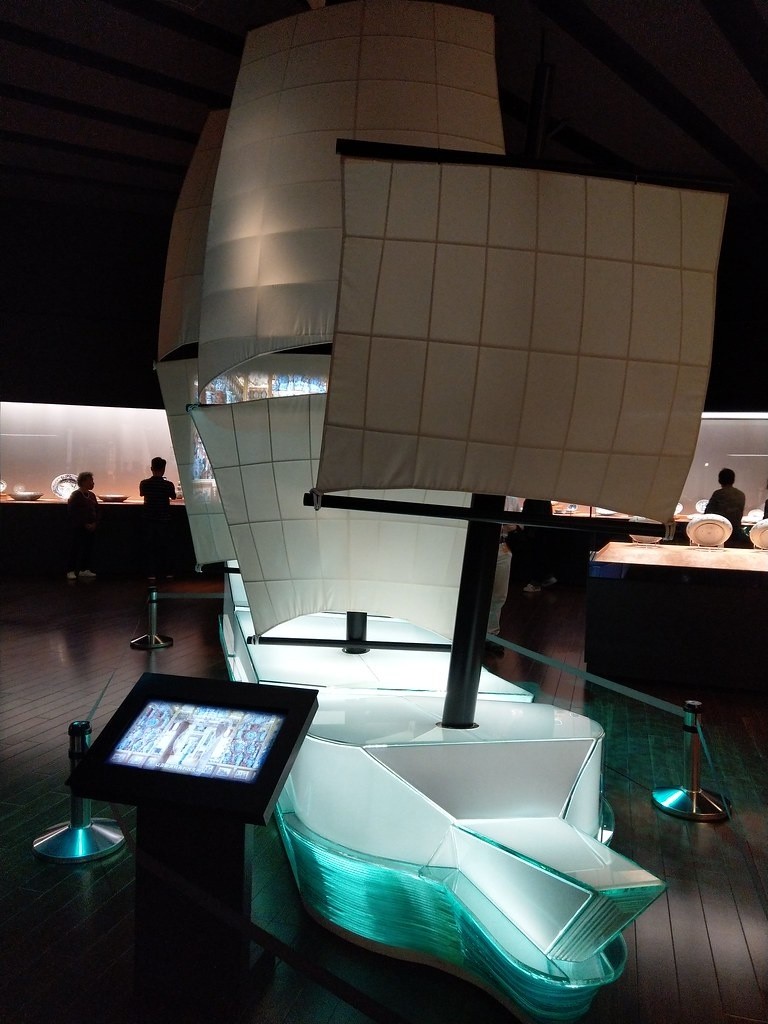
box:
[139,457,176,580]
[65,472,99,579]
[520,498,557,591]
[703,466,745,548]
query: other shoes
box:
[166,572,172,577]
[79,570,96,577]
[148,575,156,579]
[67,572,76,579]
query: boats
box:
[147,3,737,1023]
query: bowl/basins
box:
[555,509,562,513]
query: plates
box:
[595,499,768,550]
